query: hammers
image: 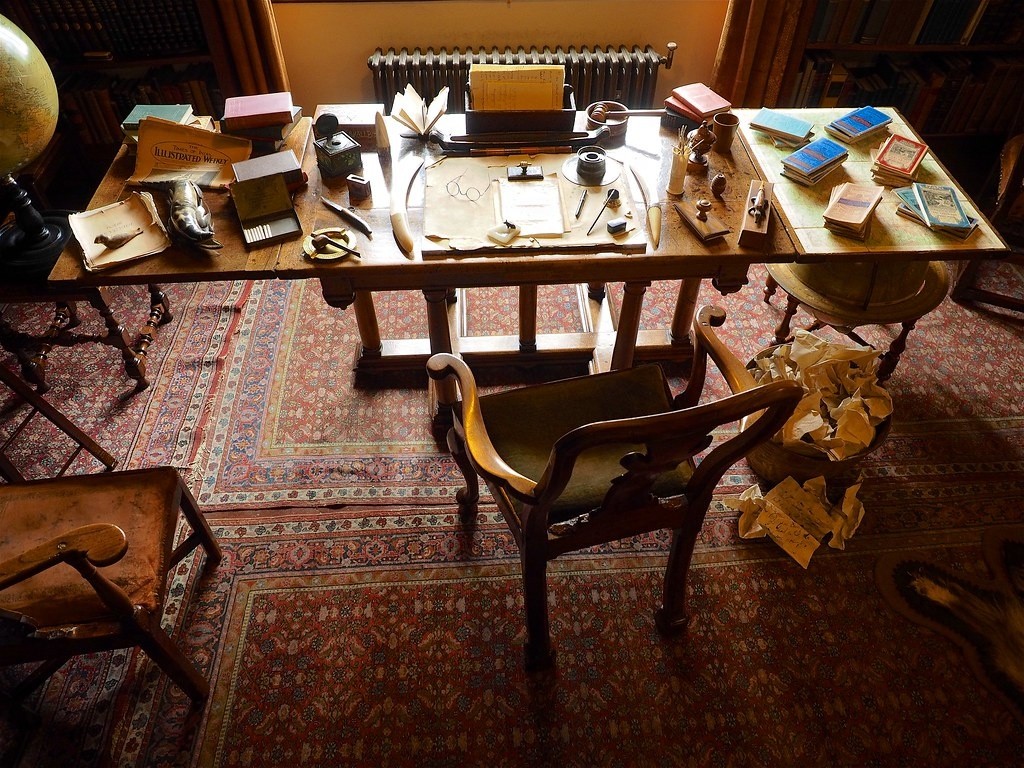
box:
[589,101,668,123]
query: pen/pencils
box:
[574,189,588,218]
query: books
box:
[121,83,449,203]
[659,82,981,241]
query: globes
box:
[0,11,86,270]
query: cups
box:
[665,146,693,195]
[712,112,740,153]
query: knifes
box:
[321,195,373,234]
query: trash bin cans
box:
[745,342,893,487]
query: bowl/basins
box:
[586,101,629,135]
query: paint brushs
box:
[586,188,617,236]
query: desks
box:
[1,205,174,389]
[50,104,1006,383]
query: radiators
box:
[366,40,680,108]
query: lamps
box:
[1,15,84,279]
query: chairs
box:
[0,468,223,712]
[425,305,804,672]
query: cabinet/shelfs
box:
[763,258,951,380]
[0,0,273,227]
[775,0,1024,140]
